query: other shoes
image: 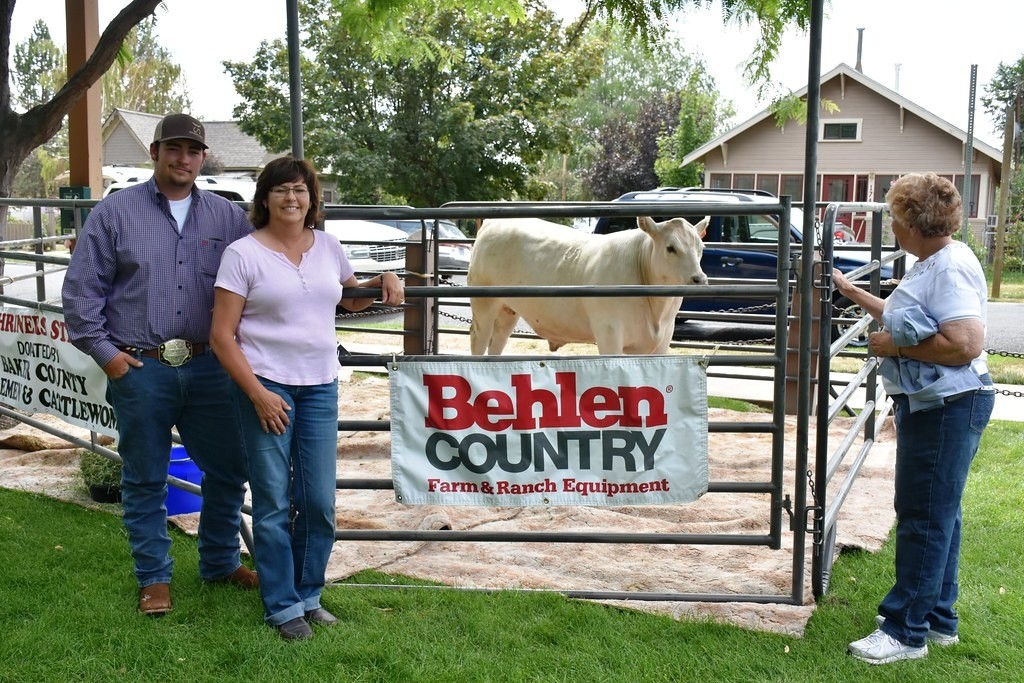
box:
[230,564,260,589]
[308,607,341,626]
[138,583,175,617]
[277,617,317,643]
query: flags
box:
[0,307,120,440]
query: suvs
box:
[324,204,472,276]
[103,175,409,315]
[593,185,909,348]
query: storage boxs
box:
[86,478,122,504]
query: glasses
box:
[269,184,310,196]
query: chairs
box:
[723,216,742,242]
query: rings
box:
[399,298,404,303]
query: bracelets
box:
[896,346,902,358]
[379,272,384,285]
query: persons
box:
[209,156,404,646]
[831,172,995,664]
[62,113,261,612]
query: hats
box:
[154,112,209,152]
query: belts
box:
[120,340,212,367]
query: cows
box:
[465,216,710,356]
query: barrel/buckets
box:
[165,447,208,516]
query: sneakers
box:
[874,613,961,646]
[847,629,930,665]
